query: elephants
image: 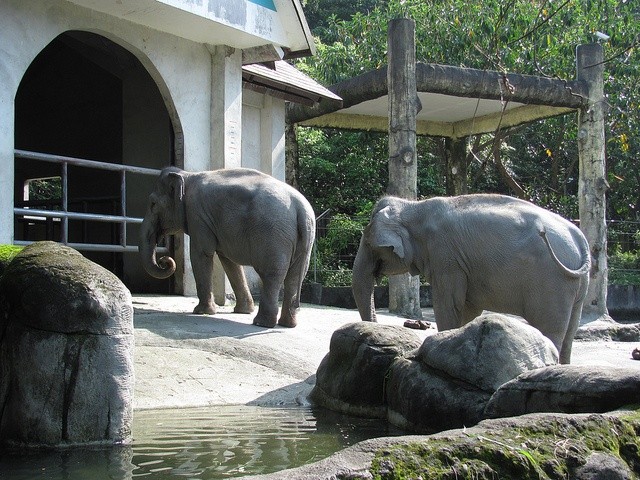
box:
[352,194,591,364]
[138,166,316,327]
